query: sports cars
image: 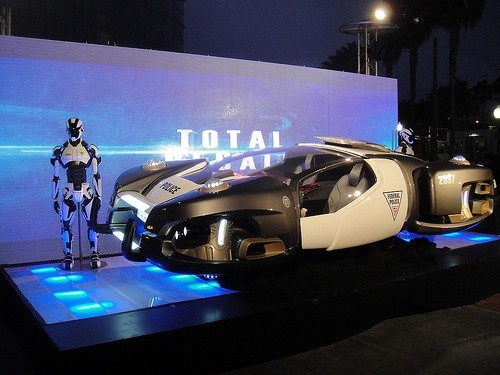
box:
[89,136,496,276]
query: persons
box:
[50,117,102,269]
[394,128,416,155]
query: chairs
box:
[284,154,318,195]
[323,162,369,214]
